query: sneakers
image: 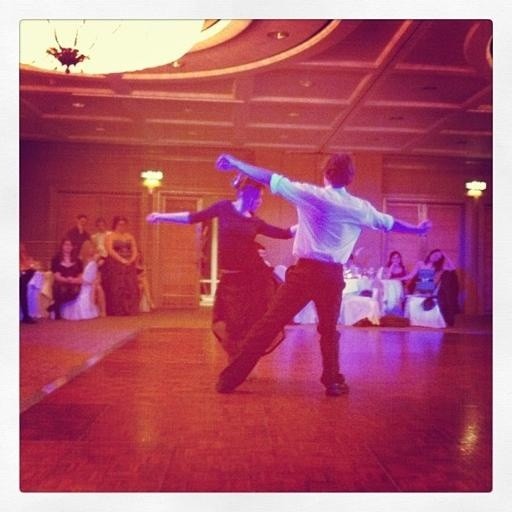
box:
[216,371,236,392]
[324,382,348,395]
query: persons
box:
[346,244,365,278]
[21,268,38,323]
[411,247,455,312]
[46,213,158,321]
[144,172,299,382]
[216,151,433,397]
[383,249,408,317]
